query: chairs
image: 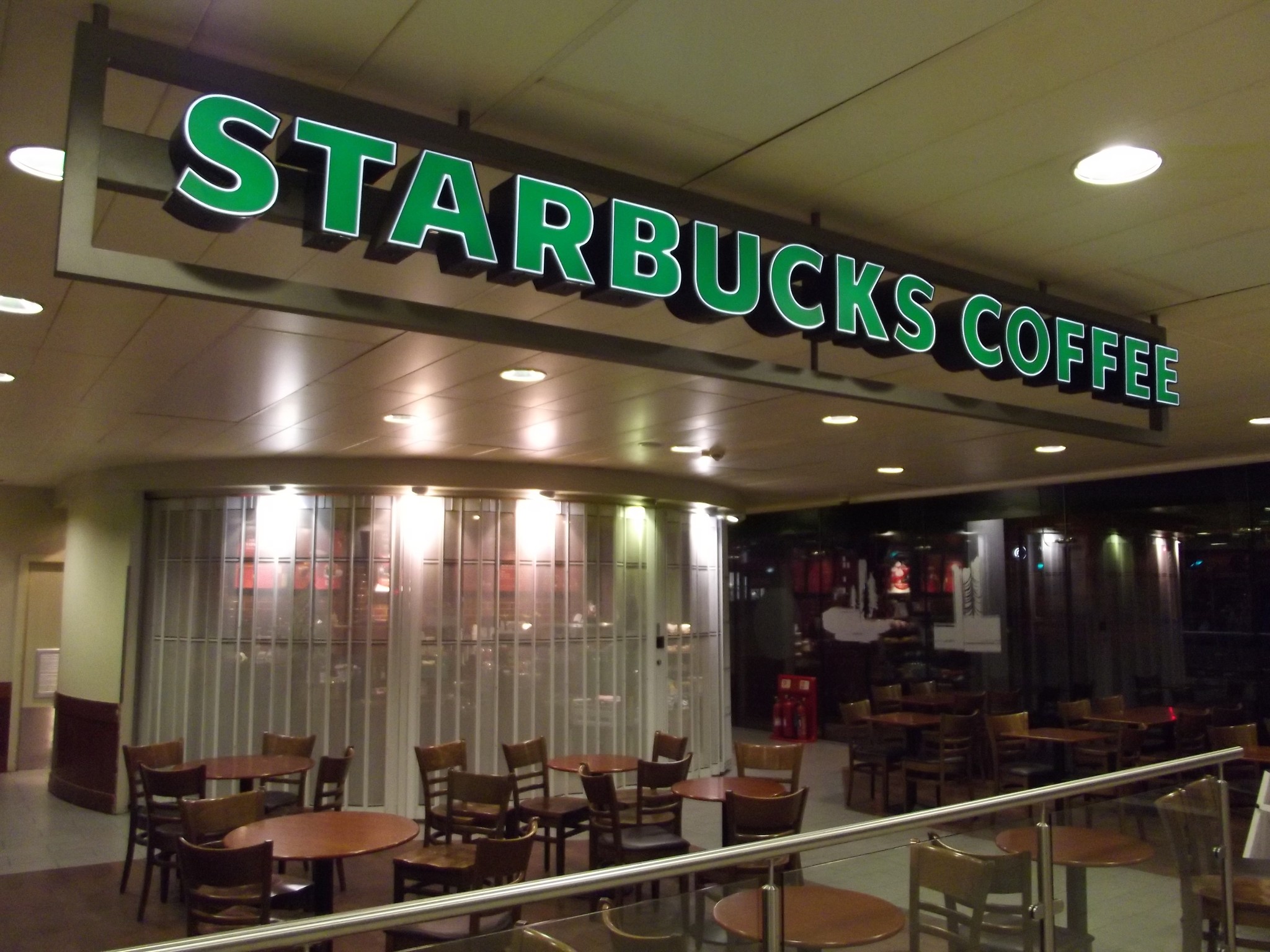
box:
[118,665,1270,952]
